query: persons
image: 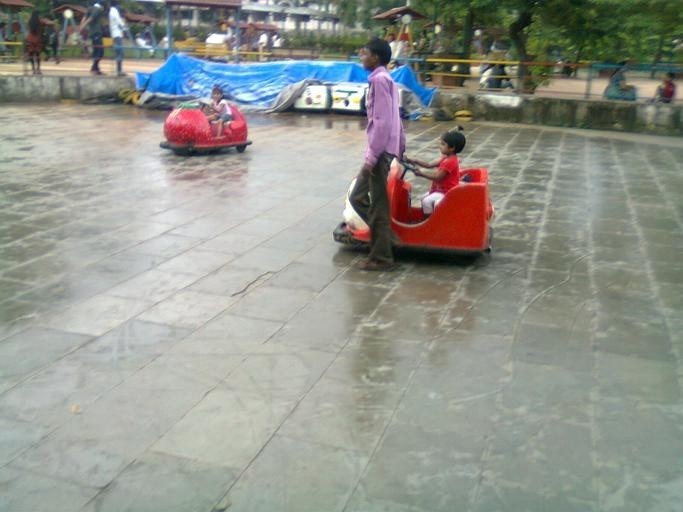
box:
[367,19,572,92]
[647,72,675,103]
[413,130,465,220]
[205,87,232,137]
[0,0,285,75]
[348,37,406,269]
[602,61,637,100]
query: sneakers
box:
[357,257,394,271]
